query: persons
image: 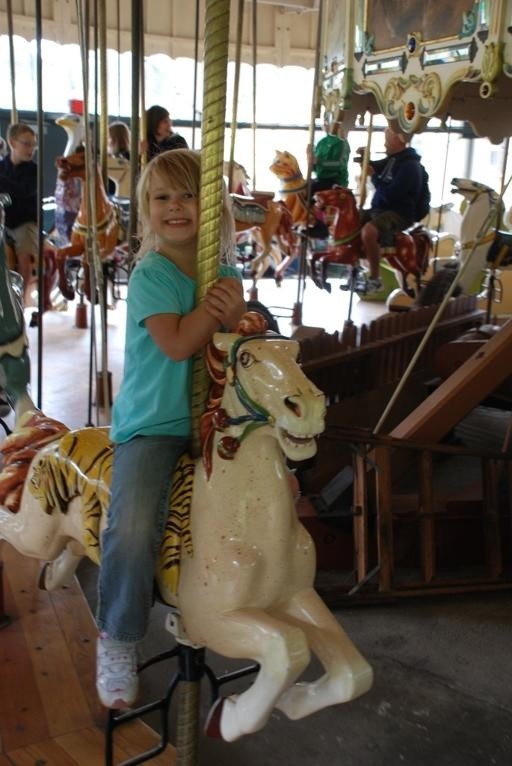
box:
[108,106,189,163]
[97,155,245,710]
[1,125,42,309]
[305,112,350,198]
[358,132,430,296]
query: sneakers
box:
[94,632,140,711]
[301,220,329,240]
[339,272,384,294]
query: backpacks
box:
[392,156,430,223]
